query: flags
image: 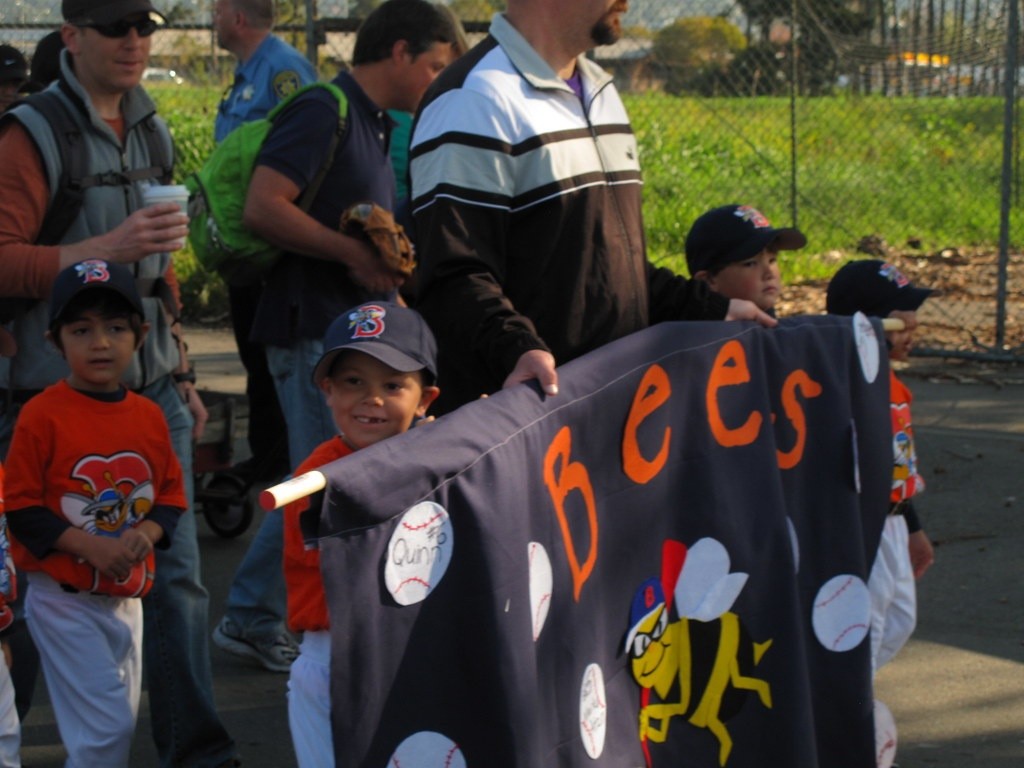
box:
[297,313,893,768]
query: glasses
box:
[70,20,156,37]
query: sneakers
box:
[212,614,301,673]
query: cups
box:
[141,187,193,246]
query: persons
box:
[687,205,808,320]
[829,259,942,768]
[405,0,778,416]
[0,0,478,768]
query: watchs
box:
[172,367,196,385]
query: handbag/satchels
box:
[182,82,350,274]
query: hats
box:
[827,260,943,320]
[61,0,168,24]
[48,258,145,326]
[0,45,26,82]
[686,204,807,275]
[19,31,66,93]
[312,301,439,389]
